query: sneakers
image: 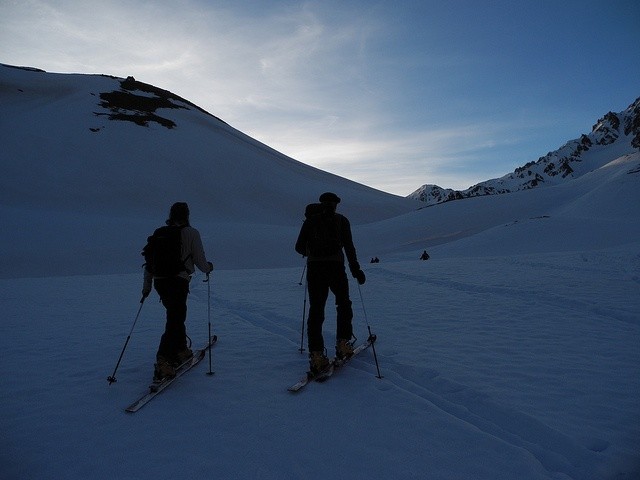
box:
[153,361,177,378]
[335,339,354,358]
[176,346,193,359]
[308,352,331,377]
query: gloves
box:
[207,262,213,272]
[142,289,149,296]
[353,270,365,284]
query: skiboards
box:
[124,335,217,412]
[287,333,376,393]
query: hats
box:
[166,202,189,225]
[319,192,340,204]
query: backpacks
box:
[141,224,191,276]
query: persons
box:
[294,189,366,372]
[370,257,376,263]
[419,250,430,261]
[140,199,213,385]
[374,256,380,263]
